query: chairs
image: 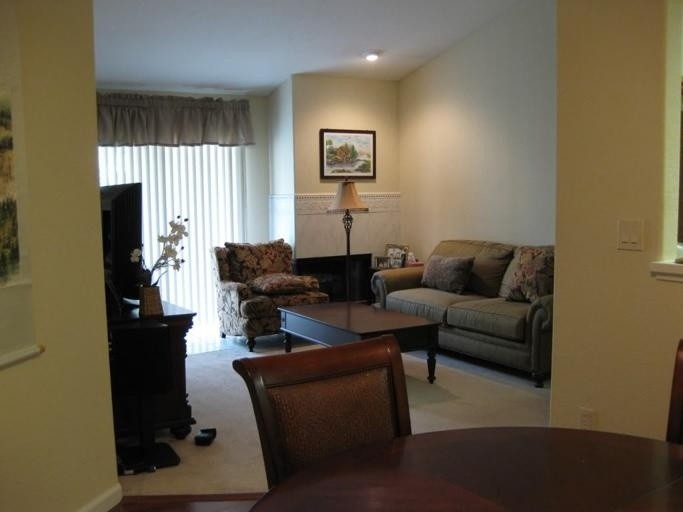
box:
[666,339,683,444]
[209,242,329,353]
[231,331,413,489]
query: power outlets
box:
[578,406,597,432]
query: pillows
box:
[224,238,320,298]
[420,238,555,303]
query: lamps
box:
[326,178,370,301]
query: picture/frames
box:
[319,128,377,180]
[374,256,390,270]
[383,243,409,268]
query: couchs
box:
[370,238,554,389]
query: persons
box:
[387,247,404,267]
[375,257,388,269]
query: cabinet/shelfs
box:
[294,253,373,305]
[404,260,424,266]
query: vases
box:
[139,285,164,316]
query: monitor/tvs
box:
[100,182,145,321]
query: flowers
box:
[129,214,189,287]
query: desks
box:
[108,295,197,441]
[247,425,683,512]
